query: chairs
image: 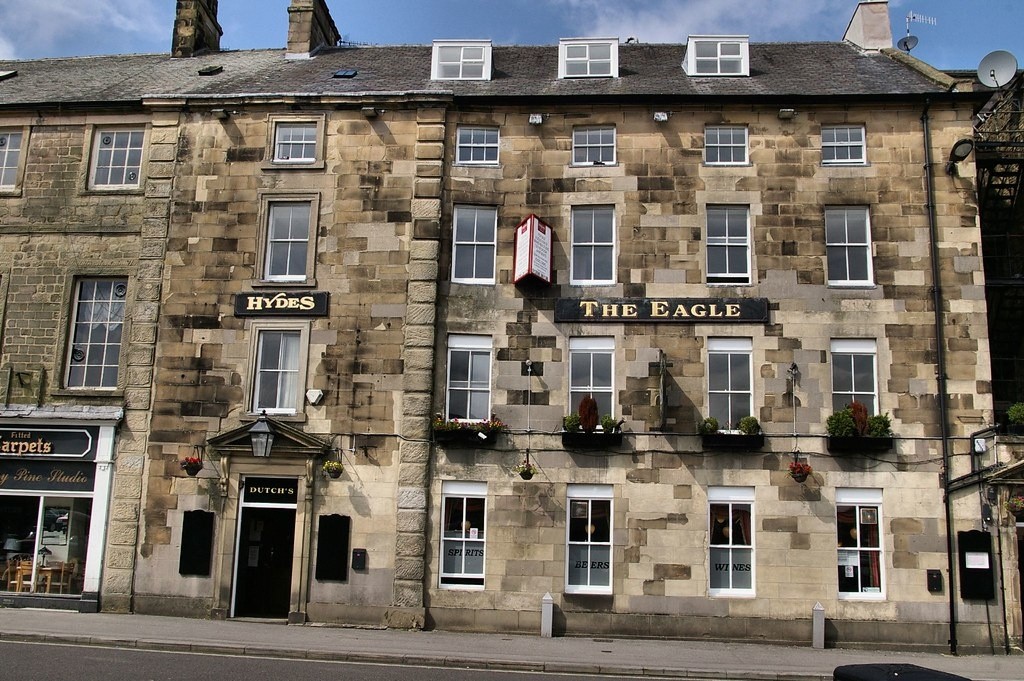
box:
[6,559,76,594]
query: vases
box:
[790,475,808,483]
[433,429,498,444]
[182,466,203,476]
[328,469,342,478]
[519,474,533,480]
[1009,510,1024,517]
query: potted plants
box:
[1006,401,1024,436]
[697,416,765,449]
[561,396,623,447]
[826,401,894,449]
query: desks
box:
[16,566,73,593]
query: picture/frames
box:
[41,506,70,545]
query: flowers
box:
[320,460,343,482]
[177,457,202,471]
[1002,496,1024,511]
[784,462,813,479]
[431,415,508,434]
[516,461,538,475]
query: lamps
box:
[461,521,471,530]
[778,109,794,119]
[851,516,858,540]
[3,538,20,553]
[246,410,277,458]
[211,109,230,119]
[653,112,670,122]
[528,113,544,126]
[723,527,729,537]
[947,139,973,175]
[585,524,595,533]
[362,106,379,116]
[38,546,52,567]
